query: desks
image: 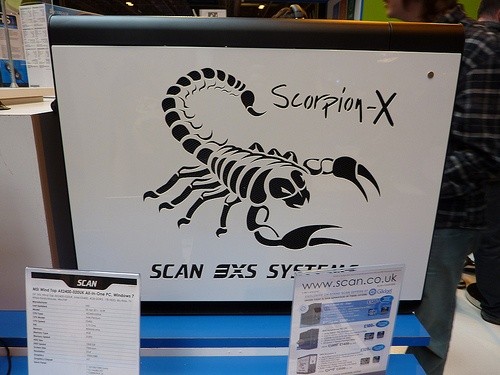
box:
[0,311,431,375]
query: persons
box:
[474,0,500,36]
[268,4,308,20]
[381,0,500,375]
